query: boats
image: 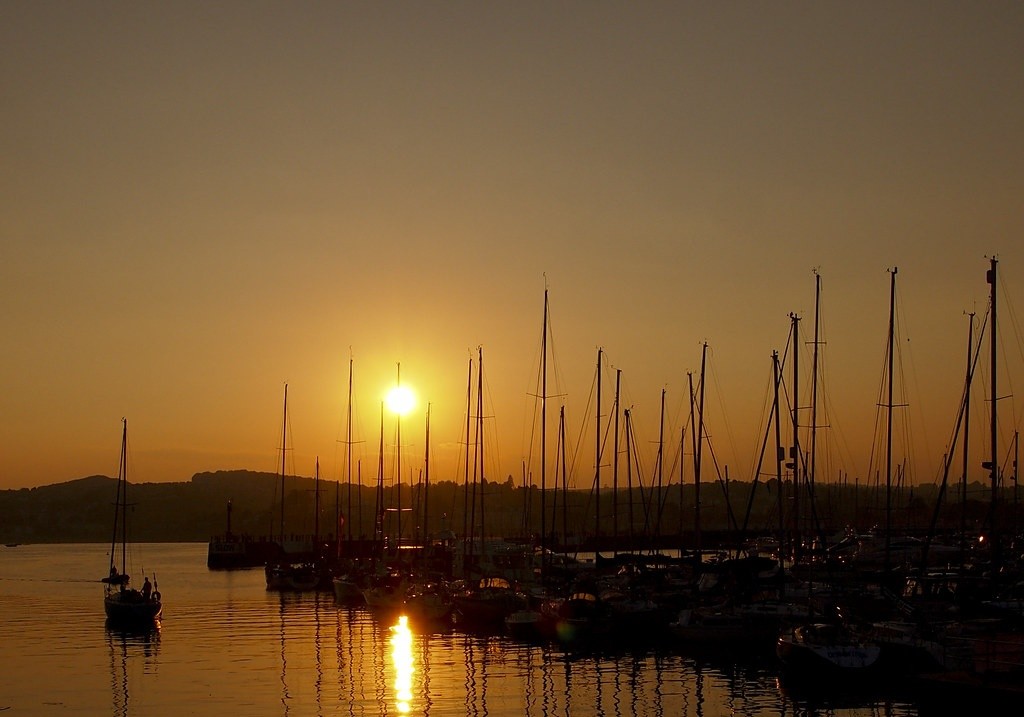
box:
[5,544,17,547]
[102,574,130,584]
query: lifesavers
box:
[151,591,161,602]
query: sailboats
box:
[207,254,1023,717]
[103,416,163,634]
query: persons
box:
[140,577,152,599]
